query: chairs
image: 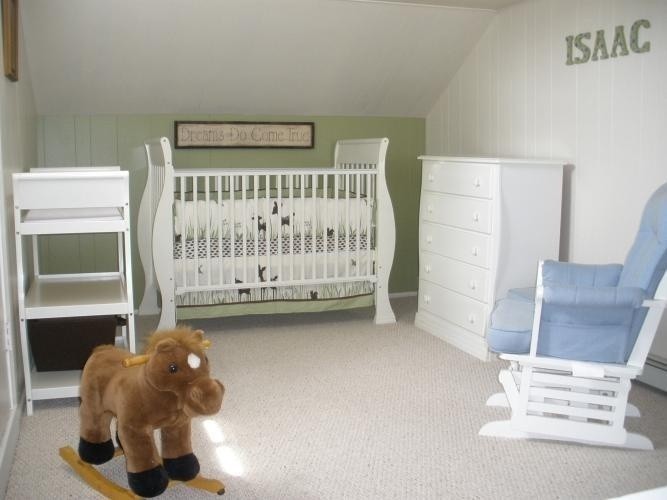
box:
[478,183,666,451]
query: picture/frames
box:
[175,122,314,149]
[2,0,19,82]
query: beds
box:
[134,137,396,334]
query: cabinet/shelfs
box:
[12,168,134,416]
[413,155,564,363]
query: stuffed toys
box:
[78,324,224,500]
